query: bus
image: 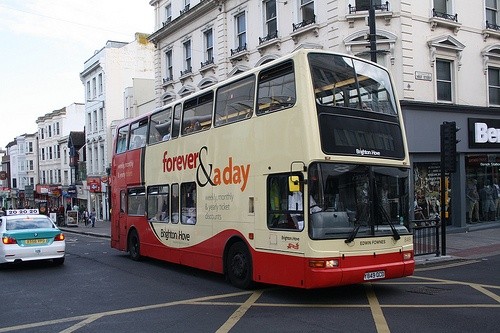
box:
[110,49,418,291]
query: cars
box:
[0,209,66,267]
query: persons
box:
[468,178,500,224]
[133,137,147,147]
[90,208,96,227]
[162,126,170,140]
[0,204,84,227]
[187,208,196,224]
[290,178,322,229]
[100,200,103,217]
[83,208,89,226]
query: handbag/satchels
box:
[82,213,85,219]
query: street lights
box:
[1,160,11,195]
